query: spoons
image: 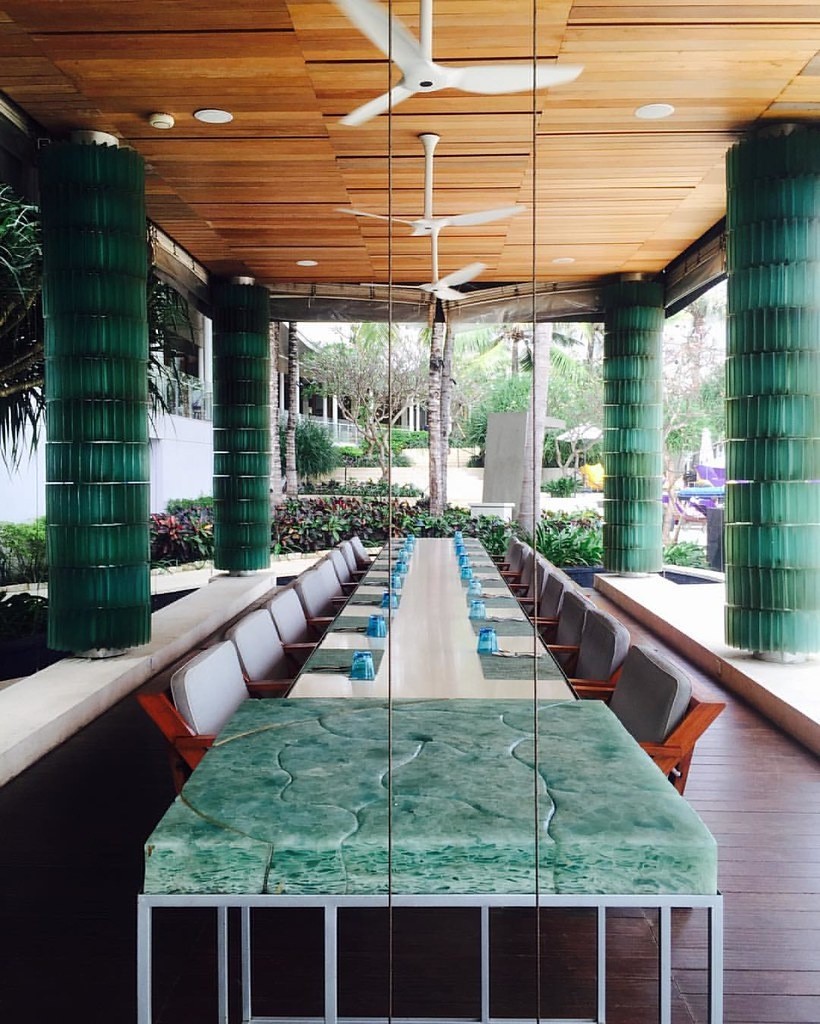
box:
[492,649,543,660]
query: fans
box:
[333,131,529,239]
[359,226,488,300]
[327,0,584,126]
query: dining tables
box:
[282,538,582,700]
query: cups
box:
[396,562,407,572]
[399,548,408,562]
[406,535,414,542]
[368,615,386,638]
[470,579,482,595]
[477,626,498,656]
[405,542,413,551]
[461,566,471,579]
[382,589,397,607]
[454,531,462,544]
[457,545,464,556]
[352,652,376,681]
[470,600,485,619]
[392,576,401,589]
[460,553,468,565]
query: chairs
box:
[531,589,598,677]
[315,558,358,613]
[138,640,296,797]
[261,588,336,666]
[296,569,349,632]
[337,540,372,584]
[326,550,368,595]
[518,571,573,635]
[350,537,378,571]
[547,608,631,699]
[574,644,727,794]
[222,609,319,697]
[490,536,554,615]
